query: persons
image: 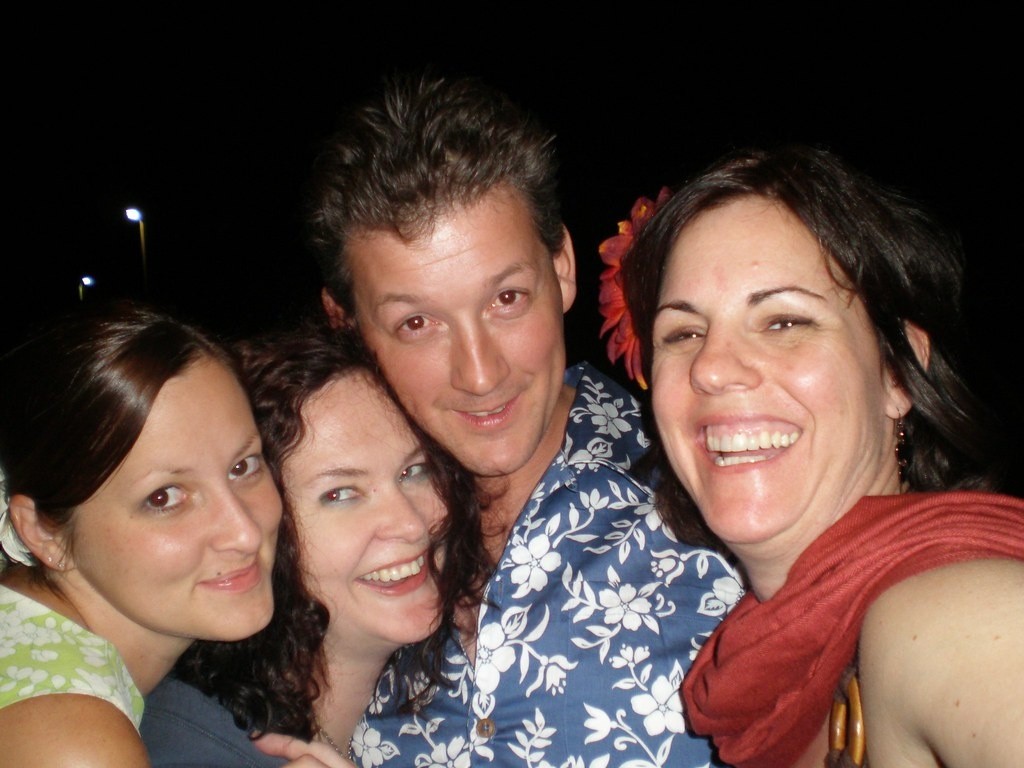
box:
[601,150,1023,768]
[137,331,505,768]
[0,305,284,768]
[313,88,744,768]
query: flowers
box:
[597,187,669,389]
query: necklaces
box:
[319,725,353,760]
